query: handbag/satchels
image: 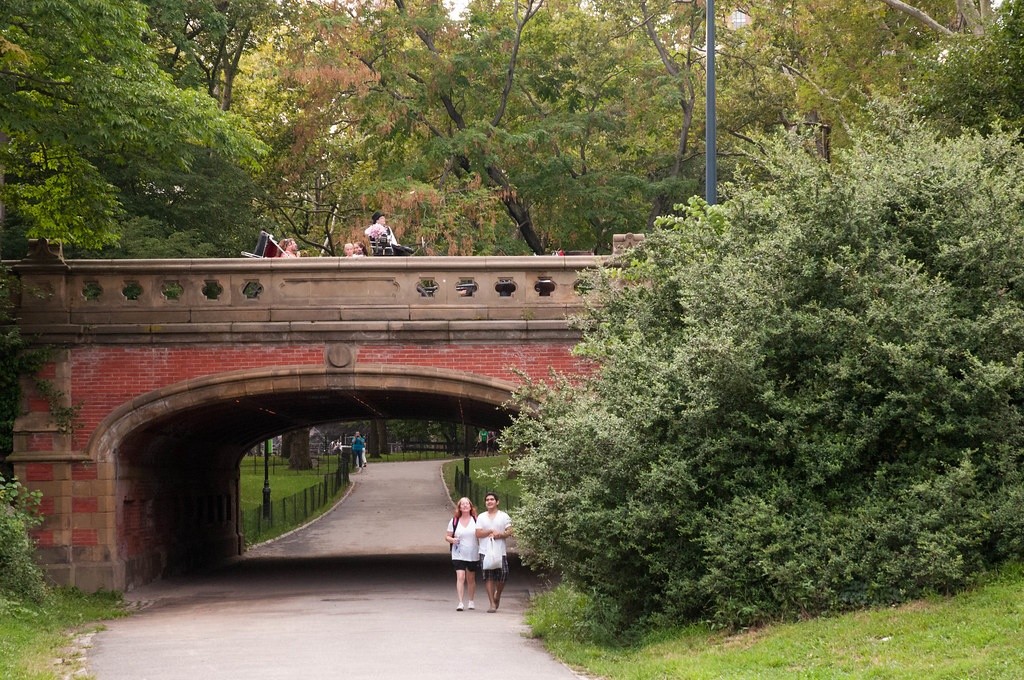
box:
[482,536,503,570]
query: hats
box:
[371,211,385,224]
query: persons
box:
[480,427,494,456]
[279,236,300,258]
[445,496,478,611]
[475,493,513,614]
[346,430,369,469]
[364,211,419,256]
[343,240,368,258]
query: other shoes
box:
[493,589,499,609]
[468,599,475,608]
[487,602,496,612]
[456,602,464,610]
